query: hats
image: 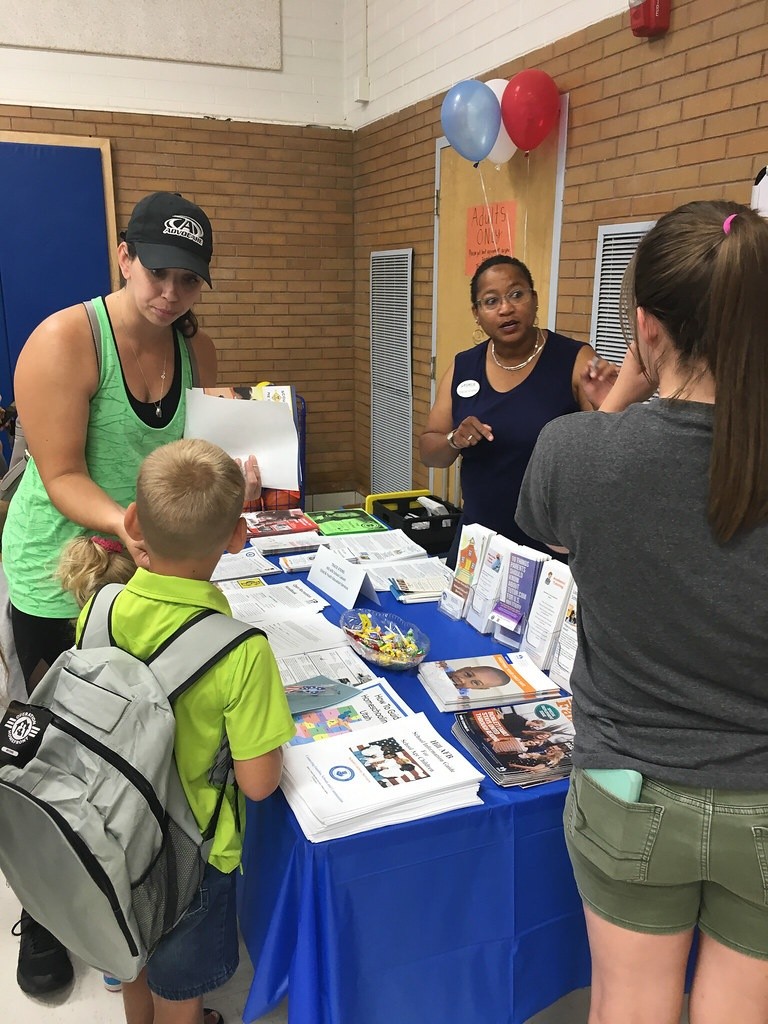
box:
[119,193,214,290]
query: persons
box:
[514,731,552,740]
[355,745,415,787]
[490,739,543,754]
[419,255,621,572]
[502,749,564,773]
[526,744,561,755]
[446,666,510,690]
[57,438,297,1024]
[1,191,262,996]
[496,712,546,737]
[307,510,369,524]
[514,199,768,1024]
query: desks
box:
[214,509,702,1024]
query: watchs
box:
[447,429,461,450]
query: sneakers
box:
[103,972,122,991]
[11,902,75,992]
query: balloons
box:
[500,69,561,158]
[440,80,501,168]
[483,78,518,172]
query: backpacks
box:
[0,579,266,982]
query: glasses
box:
[473,286,534,310]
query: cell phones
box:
[585,766,642,802]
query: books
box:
[417,651,576,789]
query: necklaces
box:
[117,291,170,418]
[491,326,546,371]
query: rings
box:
[467,434,473,441]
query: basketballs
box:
[242,499,263,513]
[263,488,299,510]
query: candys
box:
[343,613,420,668]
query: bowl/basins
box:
[340,608,431,671]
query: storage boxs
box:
[372,495,462,554]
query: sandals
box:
[203,1006,224,1024]
